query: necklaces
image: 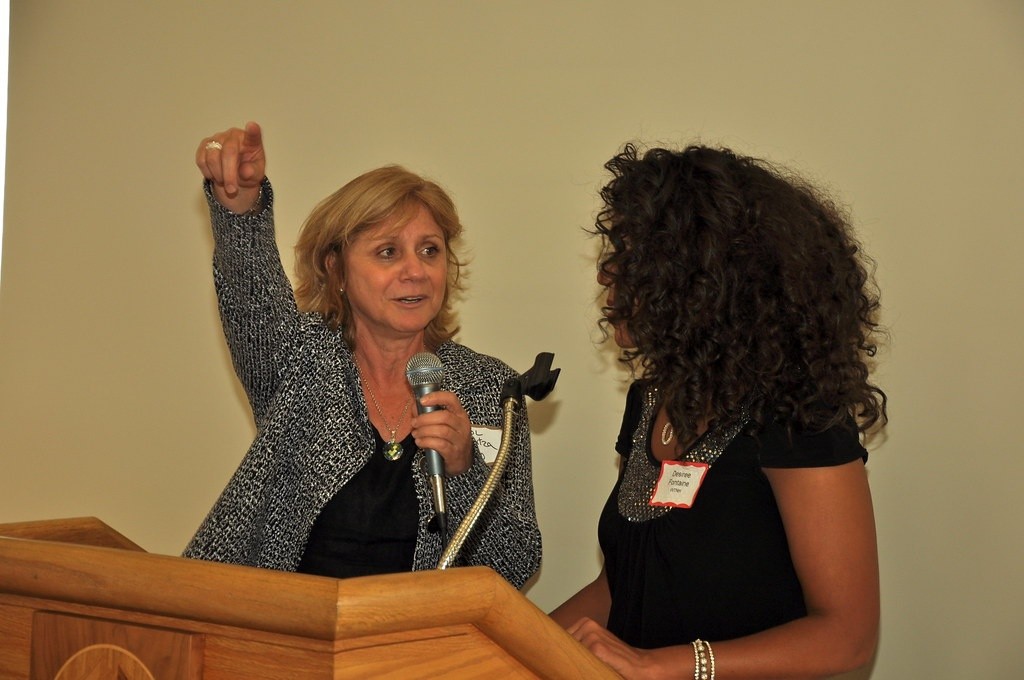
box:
[354,336,432,460]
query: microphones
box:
[406,352,448,529]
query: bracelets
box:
[690,638,716,680]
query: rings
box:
[204,138,216,149]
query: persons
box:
[180,123,542,590]
[546,143,889,680]
